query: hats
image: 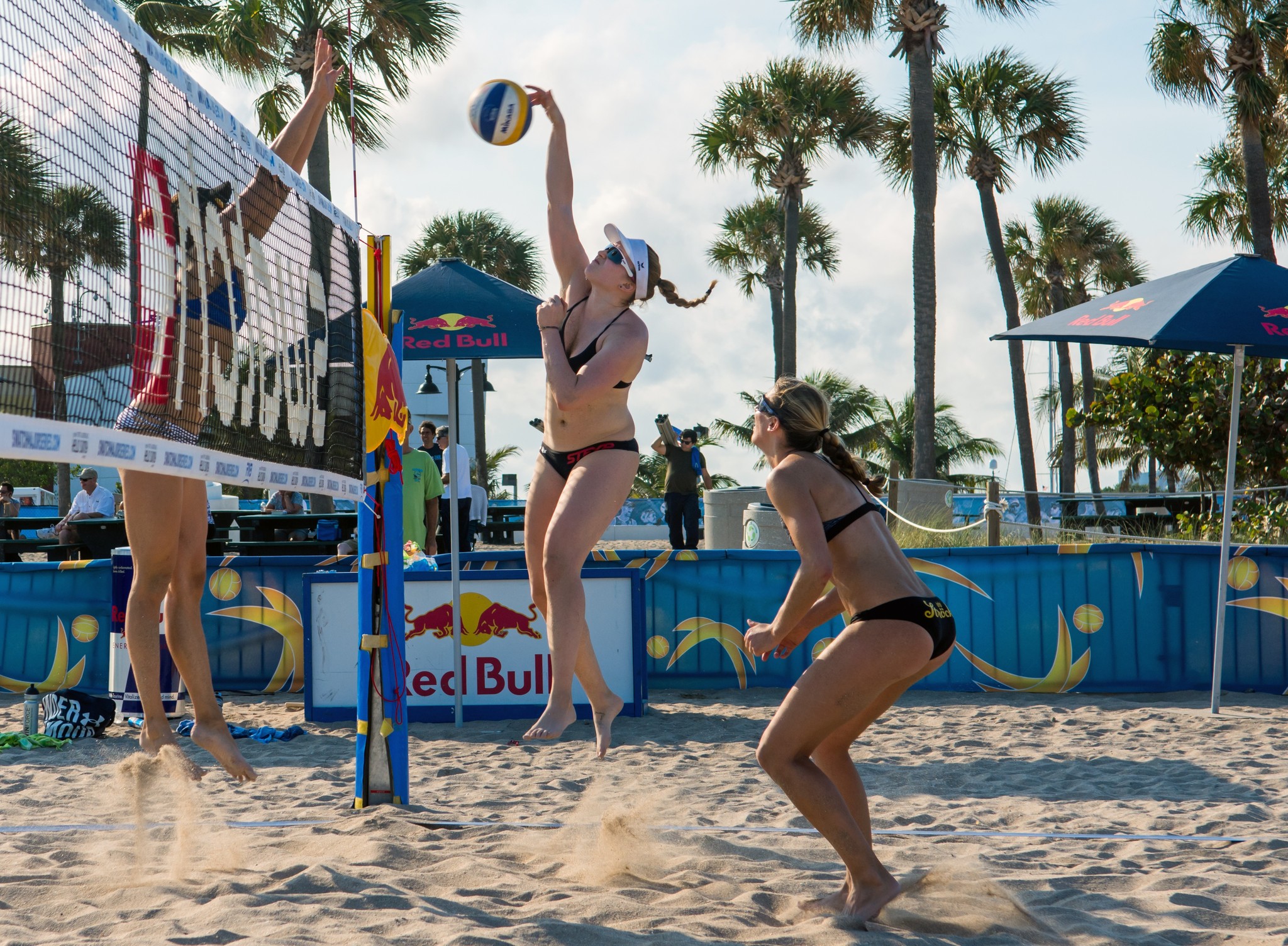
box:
[435,425,448,437]
[604,223,649,300]
[77,467,98,479]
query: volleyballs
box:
[468,79,532,146]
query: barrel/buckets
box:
[741,502,797,550]
[896,479,954,534]
[703,486,772,549]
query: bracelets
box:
[284,495,290,497]
[539,326,559,331]
[77,511,80,514]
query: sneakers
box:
[36,524,59,539]
[18,534,27,554]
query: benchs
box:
[0,522,524,550]
[1050,515,1177,528]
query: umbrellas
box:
[989,253,1288,713]
[267,257,546,728]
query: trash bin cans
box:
[741,502,796,550]
[896,478,955,532]
[108,546,186,719]
[703,486,772,549]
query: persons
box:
[743,378,958,930]
[0,481,21,540]
[206,499,225,556]
[338,409,473,555]
[522,84,717,758]
[112,29,345,782]
[119,500,124,510]
[651,429,712,550]
[55,467,114,561]
[264,490,308,542]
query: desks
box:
[67,517,125,559]
[486,506,524,544]
[0,517,64,529]
[235,513,356,555]
[1057,495,1213,535]
[209,509,261,552]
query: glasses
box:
[437,436,446,440]
[0,490,9,494]
[680,438,692,445]
[79,477,92,482]
[604,242,635,283]
[758,394,778,416]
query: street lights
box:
[415,361,497,445]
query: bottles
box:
[260,500,266,514]
[213,692,223,713]
[23,683,39,737]
[112,711,124,725]
